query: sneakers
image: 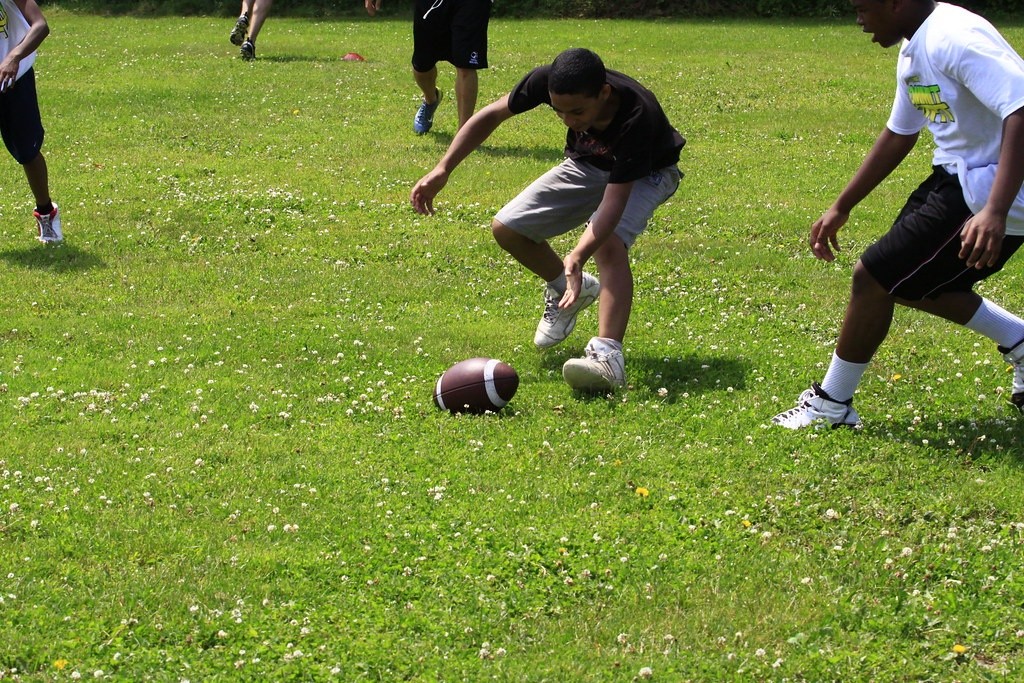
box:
[33,202,63,243]
[770,382,860,430]
[534,270,600,348]
[562,337,625,392]
[230,12,250,46]
[997,336,1024,414]
[414,86,443,133]
[240,37,255,61]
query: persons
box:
[409,48,687,389]
[229,0,271,58]
[0,0,62,244]
[758,0,1024,431]
[364,0,490,136]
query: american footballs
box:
[433,358,519,415]
